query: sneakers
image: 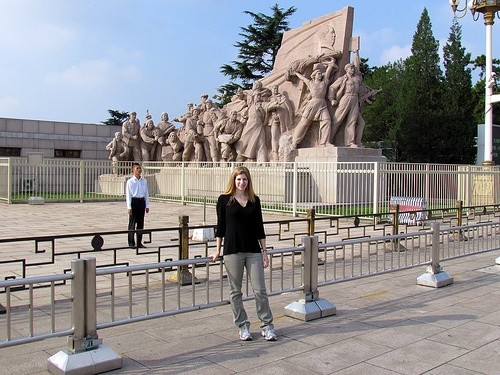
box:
[239,327,252,340]
[261,329,278,341]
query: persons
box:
[210,166,278,341]
[104,24,366,178]
[125,162,150,249]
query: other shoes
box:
[130,243,146,249]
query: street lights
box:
[449,0,500,214]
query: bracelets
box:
[263,249,267,252]
